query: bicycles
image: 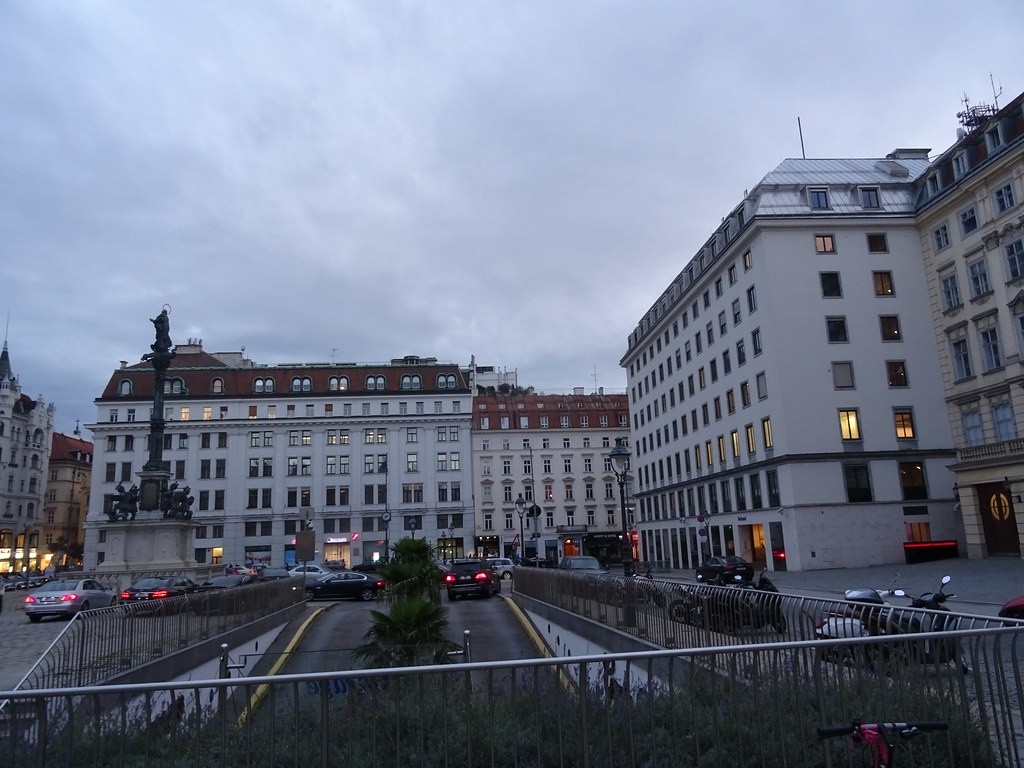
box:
[813,717,950,768]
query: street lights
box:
[448,521,456,563]
[408,515,419,540]
[515,493,527,564]
[608,435,636,577]
[441,531,447,562]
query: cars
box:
[529,557,553,567]
[193,573,280,615]
[302,569,388,602]
[23,579,119,621]
[484,557,514,580]
[0,574,50,612]
[119,574,201,614]
[257,568,290,583]
[556,555,609,575]
[288,565,333,587]
[695,555,754,582]
[231,566,257,576]
[223,566,239,576]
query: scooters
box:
[667,566,786,633]
[812,572,969,675]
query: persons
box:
[107,480,140,520]
[158,481,195,520]
[229,562,233,568]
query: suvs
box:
[446,558,502,600]
